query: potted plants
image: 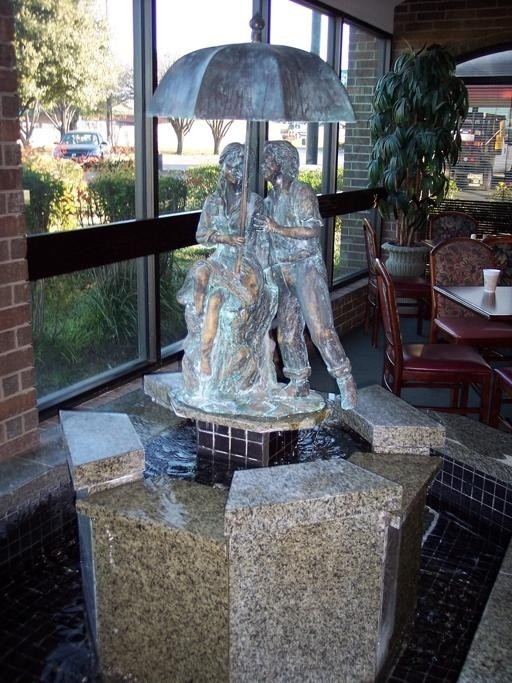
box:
[366,40,469,279]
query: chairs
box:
[363,210,512,437]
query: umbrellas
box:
[140,41,360,277]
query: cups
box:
[482,269,501,294]
[471,234,477,240]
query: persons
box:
[262,138,359,414]
[178,143,267,380]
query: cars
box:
[53,129,111,168]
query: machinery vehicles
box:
[445,106,506,192]
